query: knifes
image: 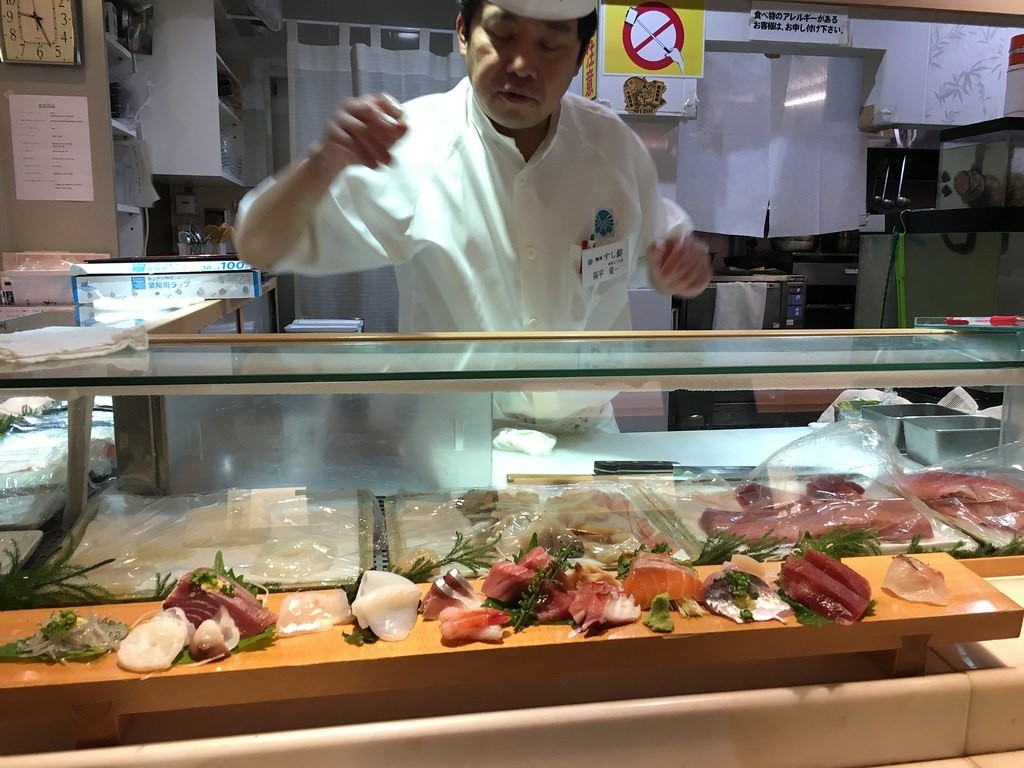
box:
[594,461,829,474]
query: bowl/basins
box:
[709,252,718,265]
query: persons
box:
[238,0,714,432]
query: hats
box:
[488,0,596,21]
